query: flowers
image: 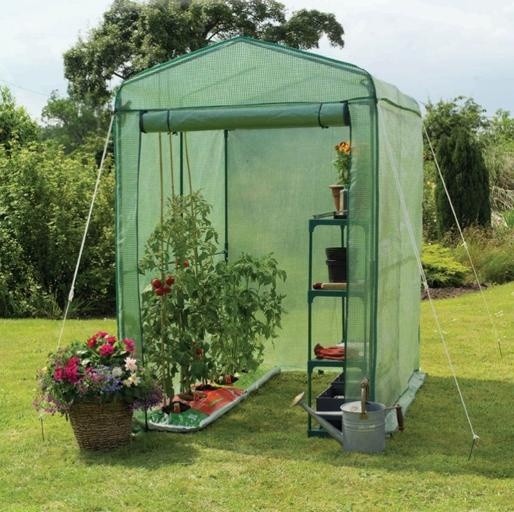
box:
[333,140,352,184]
[31,332,162,420]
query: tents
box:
[113,36,424,418]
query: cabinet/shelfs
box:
[307,213,368,439]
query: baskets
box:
[68,402,134,452]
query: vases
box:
[326,184,350,216]
